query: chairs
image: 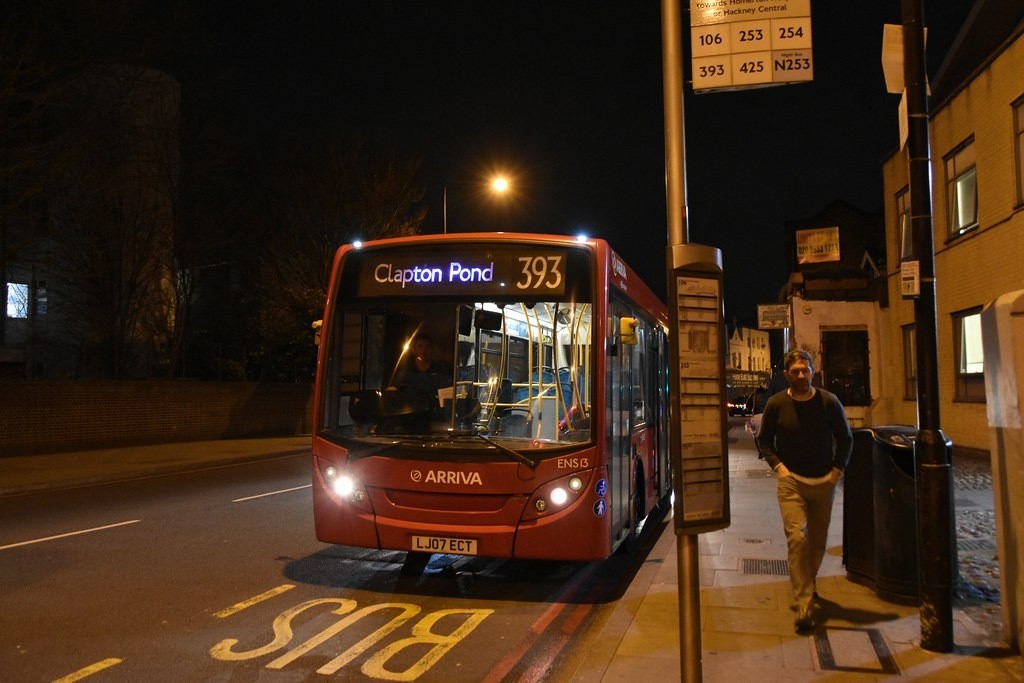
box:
[462,364,589,420]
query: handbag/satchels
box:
[745,417,756,434]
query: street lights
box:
[443,174,509,234]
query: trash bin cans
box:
[842,426,958,605]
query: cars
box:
[726,395,753,417]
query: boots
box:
[754,437,765,459]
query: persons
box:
[746,377,775,461]
[757,350,854,635]
[387,334,444,394]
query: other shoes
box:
[793,597,831,635]
[808,583,819,600]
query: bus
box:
[307,229,670,567]
